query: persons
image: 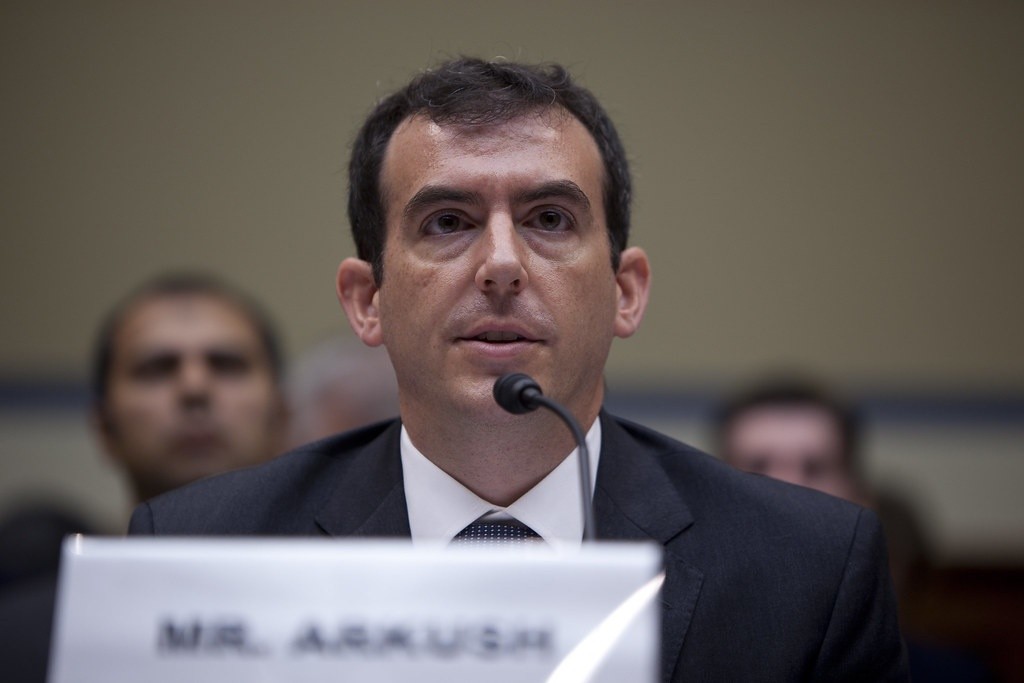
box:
[722,384,855,500]
[125,57,900,683]
[92,271,281,499]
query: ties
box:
[451,520,541,545]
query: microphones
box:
[493,370,595,540]
